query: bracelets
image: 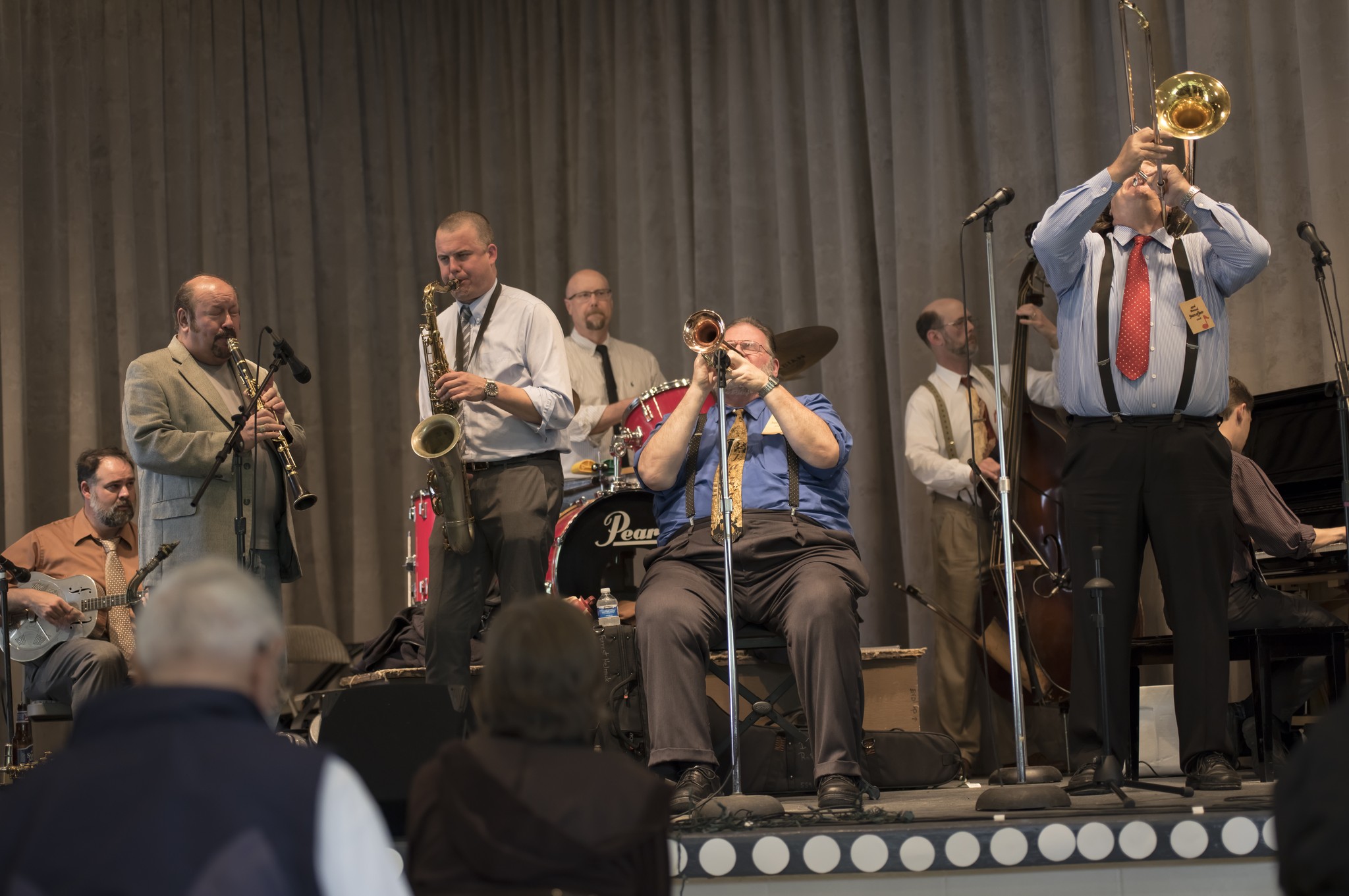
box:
[1179,185,1201,212]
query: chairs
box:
[280,625,353,747]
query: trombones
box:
[1120,0,1232,240]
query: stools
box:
[708,627,880,799]
[1126,625,1349,782]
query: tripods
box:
[1058,548,1194,807]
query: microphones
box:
[713,348,731,369]
[0,554,31,583]
[264,325,312,384]
[1296,221,1333,265]
[963,186,1015,225]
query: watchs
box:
[758,374,779,399]
[481,377,499,402]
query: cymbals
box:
[765,325,838,380]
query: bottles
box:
[596,588,620,627]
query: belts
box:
[465,451,560,470]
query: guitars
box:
[1,571,153,662]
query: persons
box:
[409,596,676,896]
[556,268,669,480]
[634,315,870,812]
[0,554,408,896]
[120,274,308,731]
[1029,125,1272,794]
[419,211,575,717]
[906,298,1059,780]
[1164,376,1348,775]
[0,448,150,735]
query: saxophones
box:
[410,277,476,556]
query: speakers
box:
[317,684,477,842]
[1273,689,1349,896]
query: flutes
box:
[225,337,317,511]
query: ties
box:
[961,375,999,450]
[96,535,137,662]
[453,305,473,453]
[1115,236,1153,380]
[595,343,620,436]
[710,409,747,546]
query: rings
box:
[1032,312,1036,318]
[259,424,266,433]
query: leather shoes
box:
[1184,753,1243,788]
[817,775,861,807]
[669,763,722,812]
[1068,756,1110,795]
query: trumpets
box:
[683,310,746,373]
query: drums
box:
[545,487,663,604]
[620,377,719,456]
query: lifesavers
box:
[411,488,440,606]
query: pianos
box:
[1213,378,1349,784]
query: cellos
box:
[986,222,1094,777]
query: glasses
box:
[569,289,612,301]
[935,316,977,329]
[725,340,768,354]
